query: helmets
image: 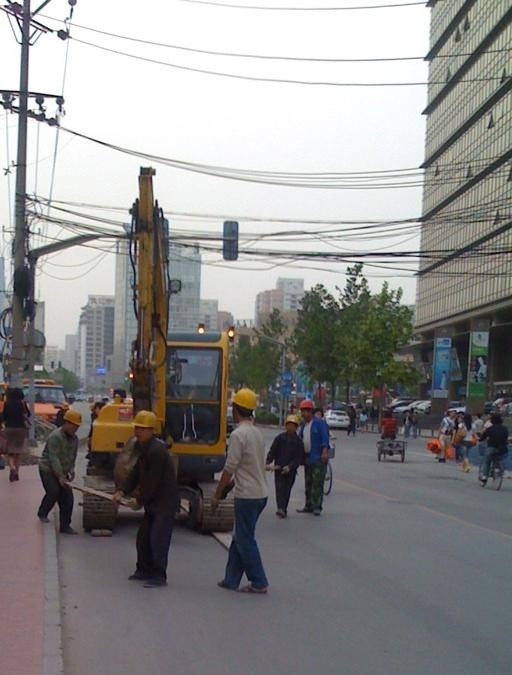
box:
[63,410,82,426]
[299,399,314,410]
[233,388,257,410]
[130,409,156,428]
[285,414,300,426]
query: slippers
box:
[241,583,268,593]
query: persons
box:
[440,371,447,390]
[474,354,488,382]
[112,410,179,590]
[295,399,329,517]
[310,407,336,444]
[208,388,269,595]
[345,405,419,441]
[265,412,303,519]
[37,409,81,536]
[434,391,512,483]
[1,387,32,481]
[166,358,218,444]
[48,388,127,460]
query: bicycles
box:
[323,435,338,496]
[477,437,507,491]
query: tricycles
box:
[373,428,408,463]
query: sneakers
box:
[478,474,487,481]
[464,465,471,472]
[9,469,15,482]
[59,526,78,535]
[296,506,323,516]
[38,511,50,522]
[13,471,18,480]
[142,579,169,589]
[218,579,237,590]
[274,507,289,519]
[128,571,149,580]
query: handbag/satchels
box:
[445,445,456,460]
[454,426,466,444]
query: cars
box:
[66,388,102,403]
[386,397,512,418]
[323,409,350,429]
[226,405,258,437]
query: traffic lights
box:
[228,327,234,342]
[196,322,206,334]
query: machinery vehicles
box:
[80,165,235,539]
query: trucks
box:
[0,378,71,425]
